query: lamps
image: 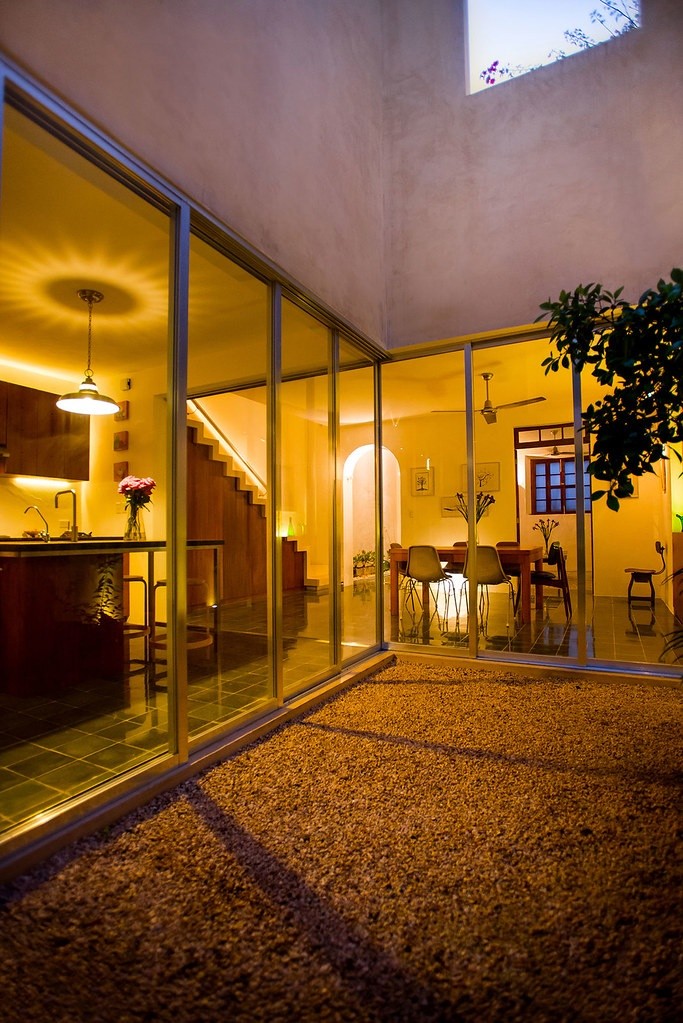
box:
[56,289,120,415]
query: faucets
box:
[55,489,78,542]
[24,506,50,542]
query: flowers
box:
[533,519,559,541]
[118,475,156,512]
[453,492,496,523]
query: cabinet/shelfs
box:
[0,381,90,482]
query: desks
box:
[387,546,543,624]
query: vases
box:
[124,509,146,541]
[543,542,549,558]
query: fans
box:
[431,373,547,425]
[545,430,575,455]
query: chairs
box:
[623,541,666,633]
[390,541,572,641]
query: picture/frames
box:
[461,462,501,491]
[410,467,436,497]
[440,496,489,518]
